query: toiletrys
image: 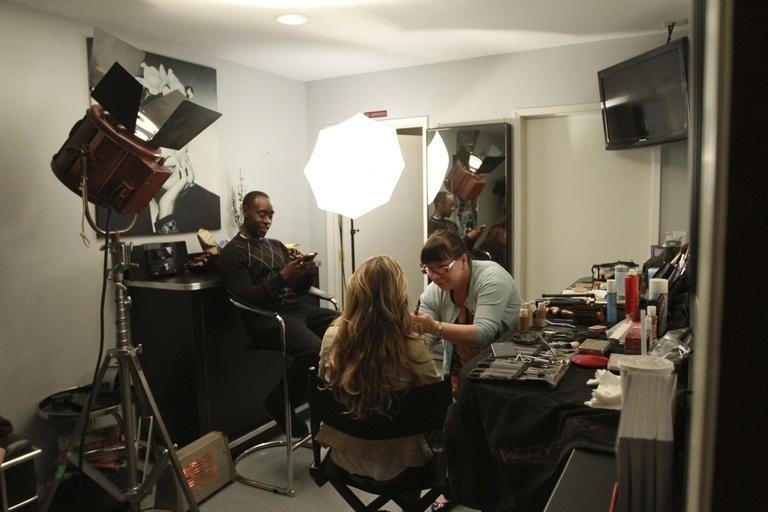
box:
[513,300,545,331]
[606,264,670,349]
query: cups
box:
[619,356,674,408]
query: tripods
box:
[41,239,202,512]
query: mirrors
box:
[422,121,515,292]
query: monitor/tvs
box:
[596,36,689,151]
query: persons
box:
[97,59,220,236]
[410,231,524,391]
[316,255,440,477]
[427,191,490,261]
[224,191,343,438]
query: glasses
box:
[421,261,456,274]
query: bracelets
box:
[434,321,442,337]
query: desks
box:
[459,312,639,512]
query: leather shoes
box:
[432,502,454,512]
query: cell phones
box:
[478,224,487,230]
[302,252,318,261]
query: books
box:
[617,372,678,511]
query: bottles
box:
[605,263,669,342]
[518,299,547,329]
